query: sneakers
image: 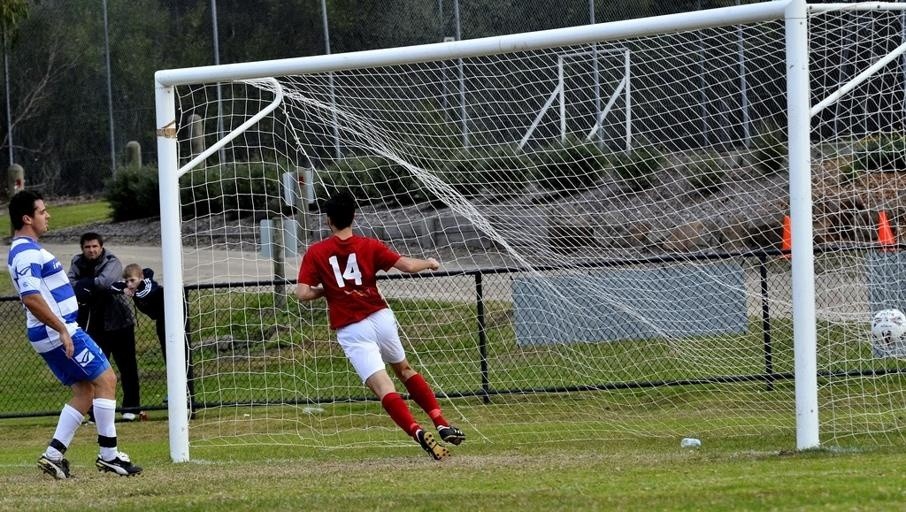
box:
[437,424,466,446]
[37,455,75,480]
[122,412,136,421]
[96,450,143,476]
[418,430,451,461]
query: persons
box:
[296,194,465,460]
[7,188,143,480]
[66,233,140,421]
[112,263,167,404]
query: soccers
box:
[871,308,905,343]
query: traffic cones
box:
[780,215,792,259]
[878,212,897,253]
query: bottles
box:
[303,407,327,416]
[681,437,701,448]
